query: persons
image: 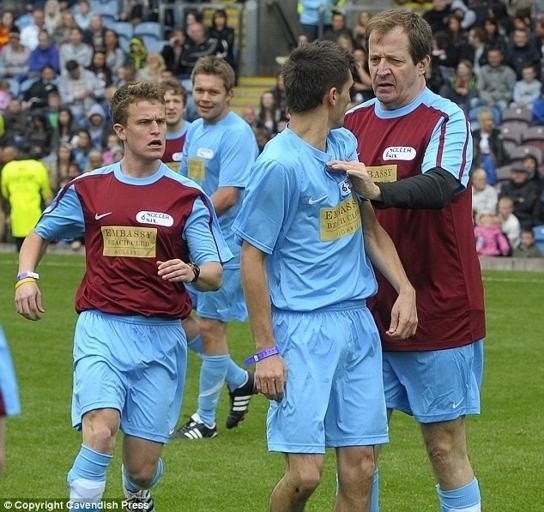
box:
[0,328,22,466]
[324,8,487,510]
[166,53,259,442]
[232,39,418,512]
[13,82,233,512]
[0,1,542,264]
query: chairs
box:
[74,0,196,121]
[455,98,543,257]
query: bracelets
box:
[14,278,36,288]
[244,345,280,366]
[13,271,39,281]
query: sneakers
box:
[171,415,218,439]
[225,371,255,429]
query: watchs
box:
[189,263,200,282]
